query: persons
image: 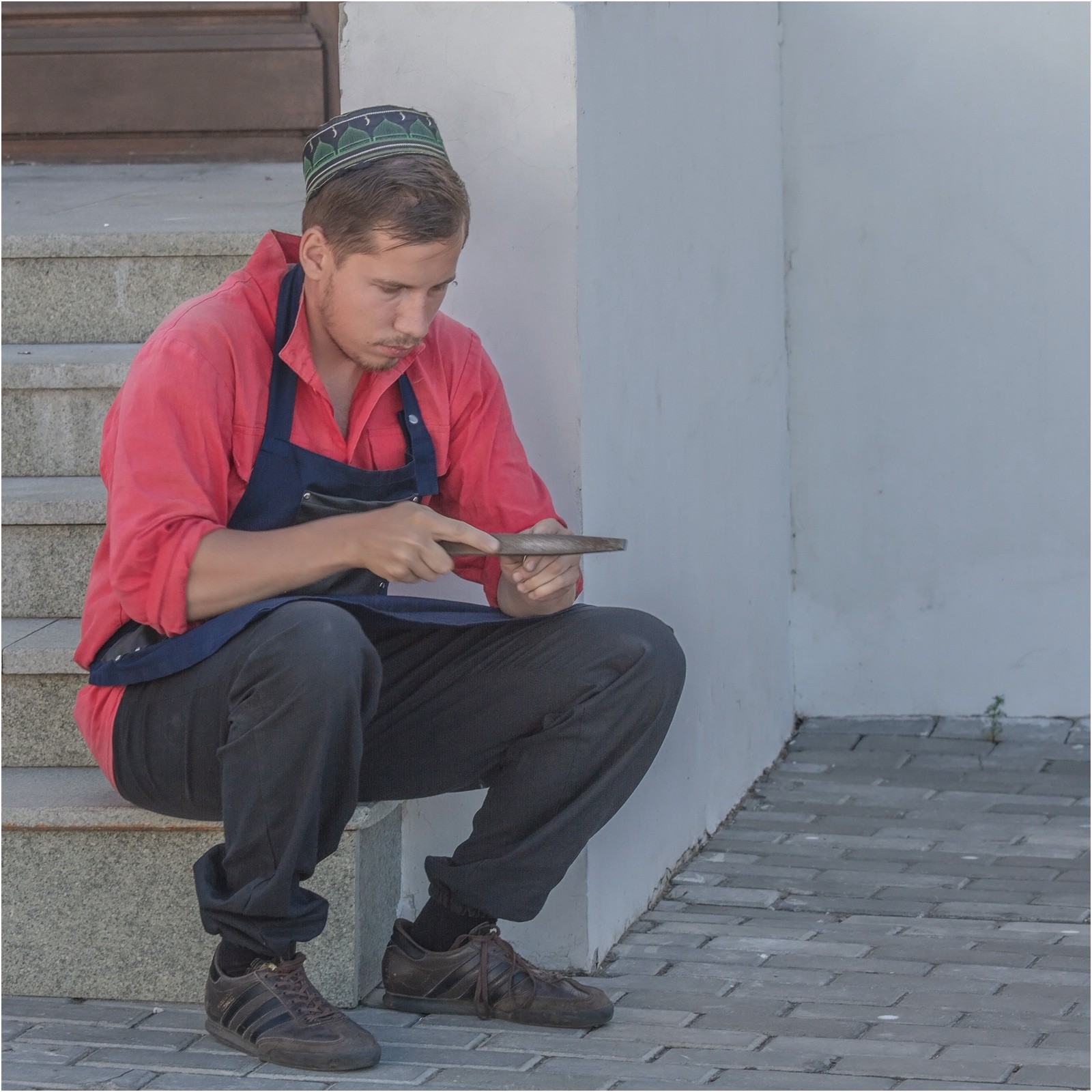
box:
[72,105,686,1071]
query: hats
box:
[300,105,450,203]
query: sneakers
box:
[383,913,614,1030]
[205,939,381,1074]
